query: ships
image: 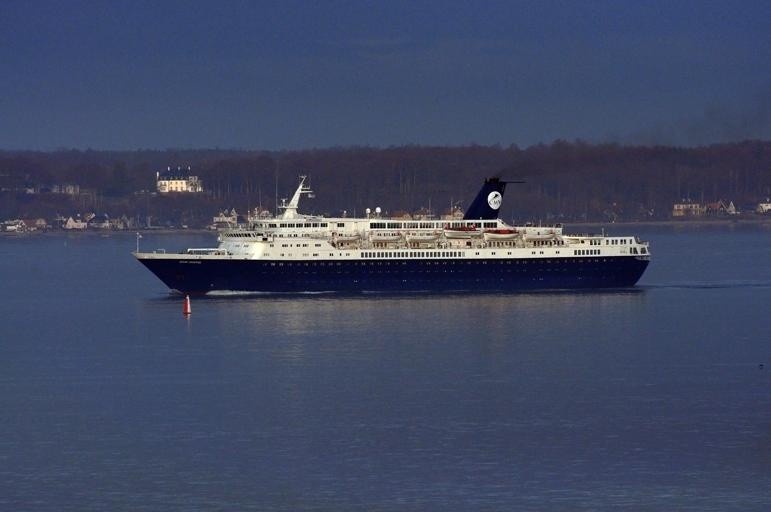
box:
[131,175,653,295]
